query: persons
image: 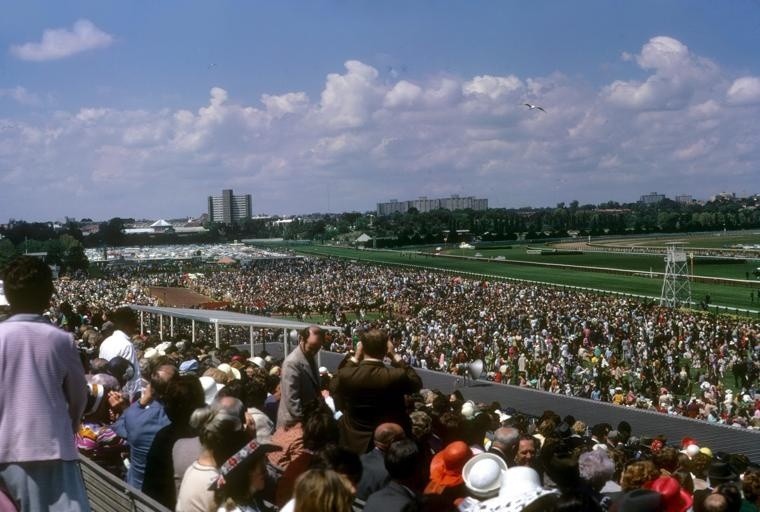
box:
[0,239,760,510]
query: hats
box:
[218,363,241,380]
[198,376,225,404]
[679,436,712,457]
[617,476,693,512]
[429,441,562,512]
[207,431,283,491]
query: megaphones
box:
[283,330,298,344]
[465,359,483,379]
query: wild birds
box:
[520,104,547,111]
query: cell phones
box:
[636,451,642,458]
